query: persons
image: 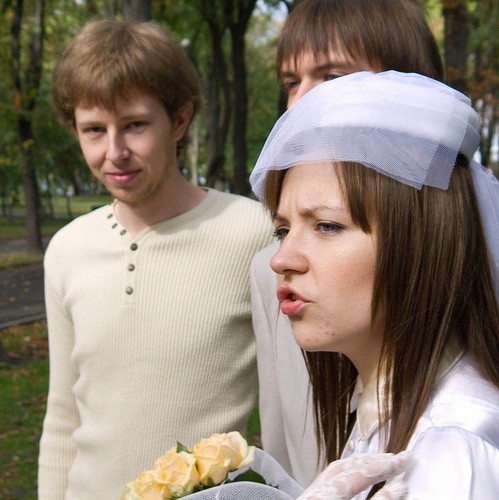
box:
[248,0,499,500]
[37,18,279,500]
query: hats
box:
[250,71,481,201]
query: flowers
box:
[120,431,255,500]
